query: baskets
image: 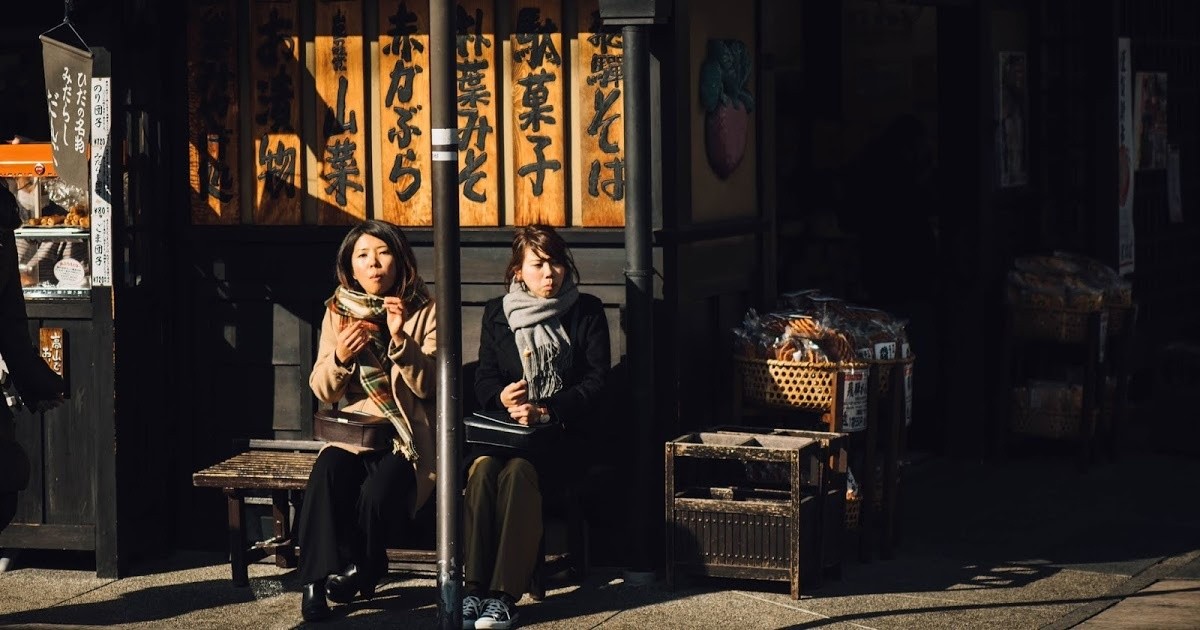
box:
[870,480,882,513]
[733,355,869,434]
[859,353,916,427]
[838,494,864,529]
[1011,399,1116,436]
[1010,306,1091,342]
[1103,304,1131,334]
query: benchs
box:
[193,444,590,600]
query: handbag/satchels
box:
[463,408,538,451]
[314,409,393,447]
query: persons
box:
[298,218,436,621]
[835,113,942,453]
[0,174,68,535]
[462,224,612,630]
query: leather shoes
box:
[302,581,330,623]
[326,578,375,603]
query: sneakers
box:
[463,597,522,629]
